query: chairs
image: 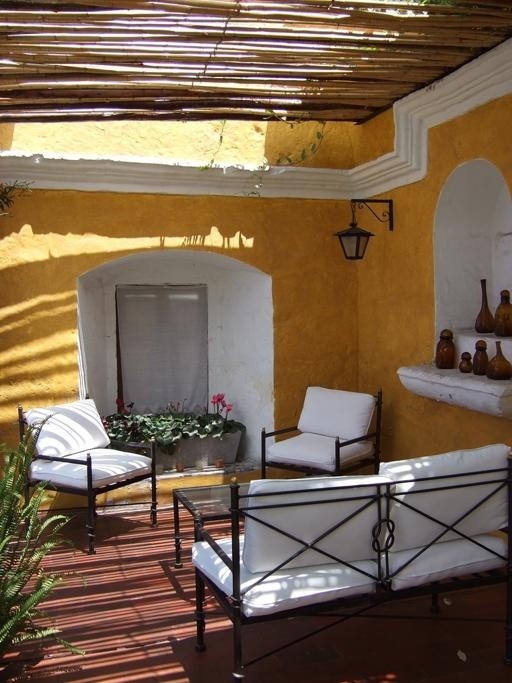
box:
[17,393,158,555]
[260,381,382,479]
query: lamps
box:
[331,197,395,259]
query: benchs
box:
[191,442,512,682]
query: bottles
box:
[435,278,512,380]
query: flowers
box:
[99,392,244,452]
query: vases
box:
[110,430,241,473]
